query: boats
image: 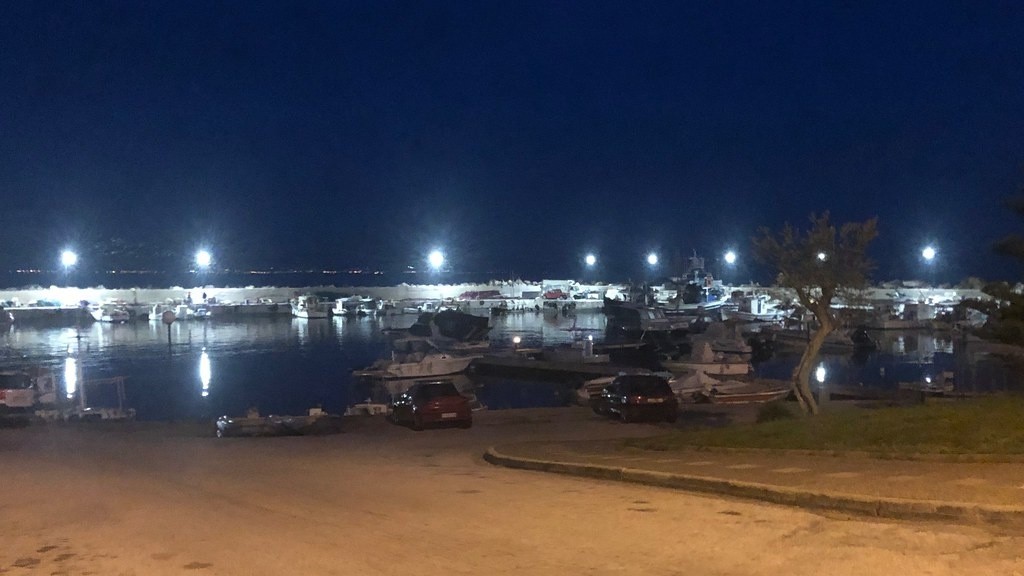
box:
[289,291,493,378]
[85,299,213,322]
[604,251,797,331]
[660,323,751,374]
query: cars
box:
[392,381,472,431]
[593,375,679,423]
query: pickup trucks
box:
[543,289,571,300]
